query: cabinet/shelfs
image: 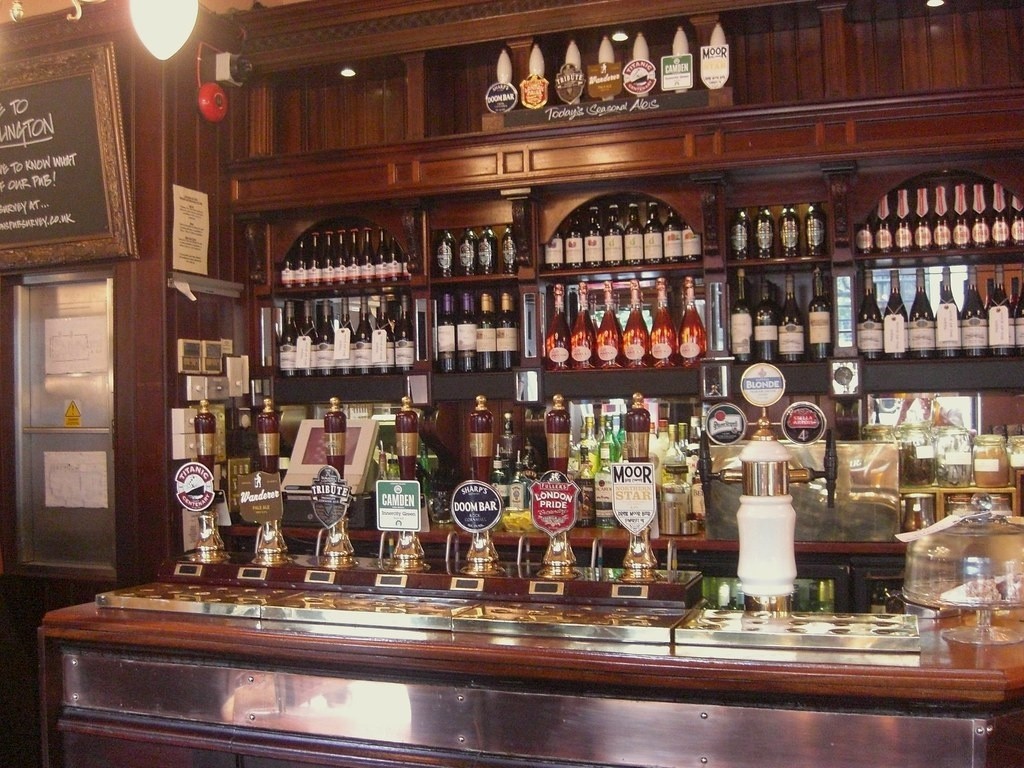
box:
[233,101,1024,441]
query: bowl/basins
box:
[500,509,539,533]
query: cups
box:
[427,489,454,524]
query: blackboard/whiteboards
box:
[0,42,139,271]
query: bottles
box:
[571,412,716,536]
[427,221,521,374]
[375,411,539,509]
[271,224,416,377]
[540,198,708,373]
[862,422,1024,533]
